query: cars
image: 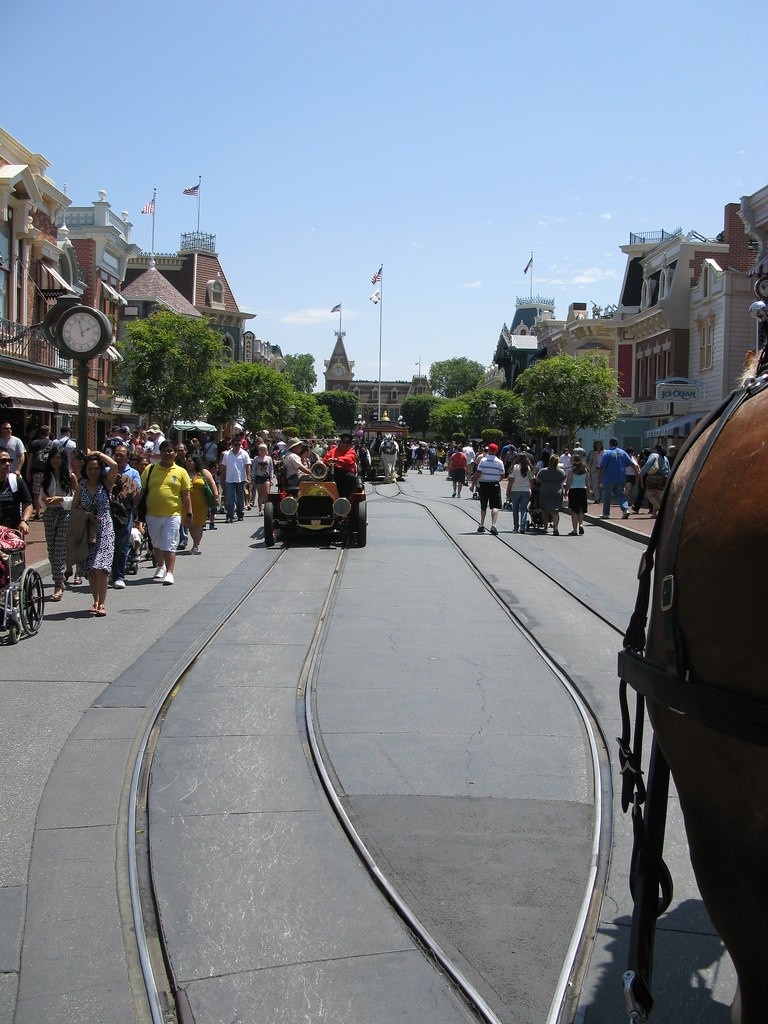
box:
[264,457,368,547]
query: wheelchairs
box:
[0,530,45,644]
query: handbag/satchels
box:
[137,489,148,523]
[648,473,667,490]
[200,470,219,510]
[33,441,53,469]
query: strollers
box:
[525,492,553,530]
[504,498,513,511]
[123,513,148,575]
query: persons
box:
[0,422,679,617]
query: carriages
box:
[361,421,409,484]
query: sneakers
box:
[163,573,174,585]
[154,565,166,578]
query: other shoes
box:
[203,524,206,531]
[477,525,585,535]
[114,580,126,589]
[74,577,82,585]
[178,543,186,549]
[33,514,41,522]
[90,602,106,617]
[260,510,264,516]
[452,493,461,498]
[600,504,657,519]
[53,586,63,601]
[192,546,201,555]
[226,516,238,523]
[210,523,217,530]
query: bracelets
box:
[215,495,219,498]
[97,451,101,457]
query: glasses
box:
[0,458,14,464]
[234,444,242,446]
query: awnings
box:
[644,411,713,440]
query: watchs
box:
[187,512,193,518]
[21,519,29,525]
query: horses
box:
[379,432,399,484]
[623,349,768,1024]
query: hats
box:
[340,434,354,443]
[145,424,164,437]
[287,438,303,450]
[111,426,121,431]
[488,444,498,452]
[61,426,73,432]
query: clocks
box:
[63,314,101,352]
[335,368,343,376]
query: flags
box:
[331,303,341,312]
[523,258,534,274]
[183,184,200,196]
[141,199,155,215]
[369,288,381,305]
[370,267,383,285]
